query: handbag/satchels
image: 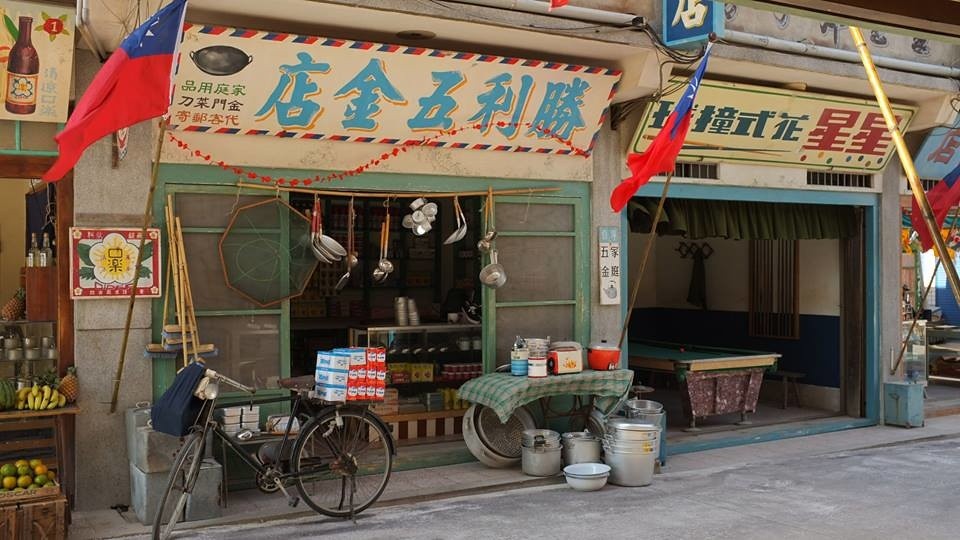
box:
[151,361,208,437]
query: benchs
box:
[764,369,807,410]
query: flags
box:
[40,0,189,183]
[610,50,714,214]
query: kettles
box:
[604,280,617,298]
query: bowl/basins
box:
[563,462,612,491]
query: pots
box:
[562,432,601,464]
[585,340,622,369]
[520,428,563,476]
[601,399,667,485]
[190,45,253,75]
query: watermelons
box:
[0,377,15,410]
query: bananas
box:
[15,385,66,410]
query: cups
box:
[528,357,547,377]
[442,363,483,378]
[511,351,529,375]
[394,296,420,326]
[7,377,40,390]
[0,333,57,360]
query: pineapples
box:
[1,288,25,321]
[59,367,80,402]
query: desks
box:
[628,338,785,433]
[457,369,636,432]
[216,430,313,508]
[0,403,79,522]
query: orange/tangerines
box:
[0,459,55,491]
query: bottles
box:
[40,233,52,266]
[514,335,527,351]
[27,232,39,267]
[6,17,40,115]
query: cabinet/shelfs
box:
[903,319,928,399]
[291,196,441,330]
[18,266,58,319]
[925,327,960,384]
[0,317,59,391]
[347,325,482,442]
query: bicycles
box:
[150,361,399,540]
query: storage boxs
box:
[314,346,434,413]
